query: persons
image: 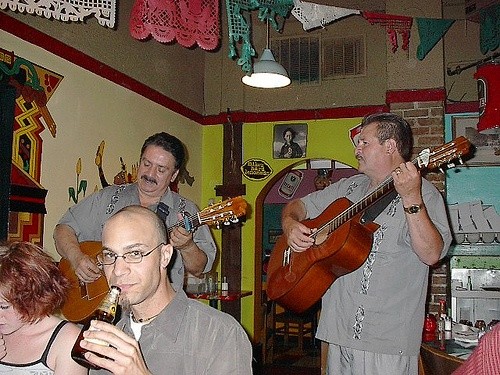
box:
[0,240,89,375]
[279,128,303,158]
[80,205,253,375]
[282,112,453,375]
[53,132,217,292]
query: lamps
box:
[241,16,291,90]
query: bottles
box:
[467,276,472,291]
[71,285,121,371]
[422,299,453,343]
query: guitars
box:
[56,195,249,323]
[265,134,473,312]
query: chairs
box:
[275,298,322,352]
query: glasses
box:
[95,242,167,265]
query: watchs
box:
[403,202,425,214]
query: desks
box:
[184,288,253,309]
[419,336,482,375]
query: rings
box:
[396,170,402,175]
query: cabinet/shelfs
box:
[446,256,500,328]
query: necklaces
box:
[130,312,159,323]
